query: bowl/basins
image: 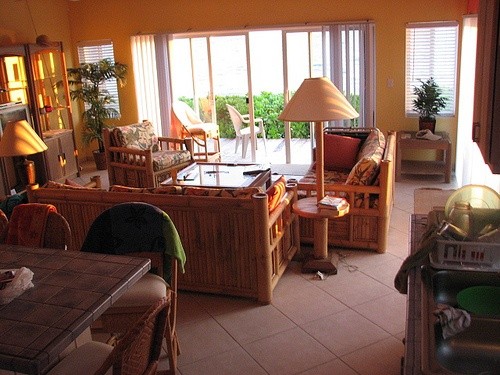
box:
[456,285,500,317]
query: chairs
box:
[226,103,267,158]
[79,202,186,375]
[48,290,173,375]
[173,99,221,163]
[5,204,71,250]
[102,120,194,191]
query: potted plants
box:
[52,58,128,171]
[413,77,449,134]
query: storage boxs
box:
[426,209,500,272]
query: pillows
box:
[323,133,360,170]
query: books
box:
[317,195,346,210]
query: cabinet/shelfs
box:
[471,0,500,174]
[0,41,48,197]
[405,206,500,375]
[395,131,451,183]
[25,40,80,183]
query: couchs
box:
[25,176,301,306]
[288,127,396,253]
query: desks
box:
[160,159,271,190]
[293,197,349,275]
[0,245,151,375]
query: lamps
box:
[279,77,360,205]
[0,120,49,190]
